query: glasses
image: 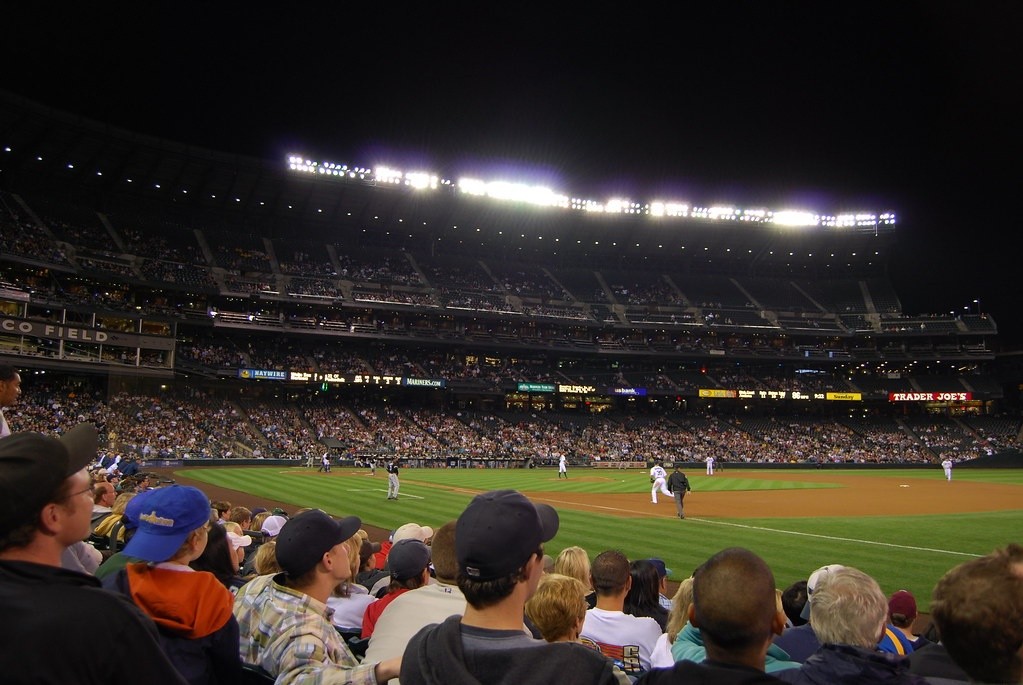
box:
[62,485,95,500]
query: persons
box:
[0,424,1023,685]
[0,223,1023,519]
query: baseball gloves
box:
[650,478,655,483]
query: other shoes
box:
[388,497,399,500]
[677,513,684,519]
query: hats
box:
[456,488,559,582]
[107,475,117,481]
[800,564,844,619]
[360,540,382,565]
[275,509,361,577]
[393,523,433,546]
[120,484,211,563]
[889,590,916,620]
[0,423,97,529]
[649,559,672,578]
[227,532,252,551]
[388,538,431,579]
[261,516,287,537]
